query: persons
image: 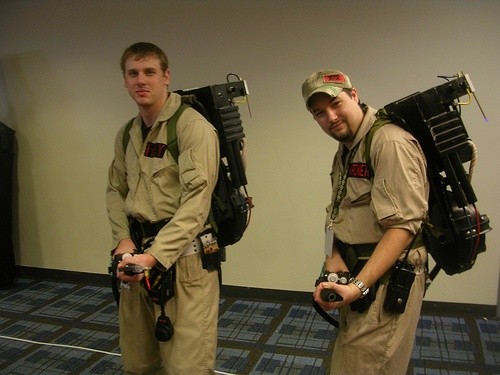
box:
[106,42,220,375]
[301,68,429,375]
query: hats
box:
[301,69,352,111]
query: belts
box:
[178,225,216,258]
[334,240,424,257]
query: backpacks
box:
[122,93,248,248]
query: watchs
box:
[348,278,369,298]
